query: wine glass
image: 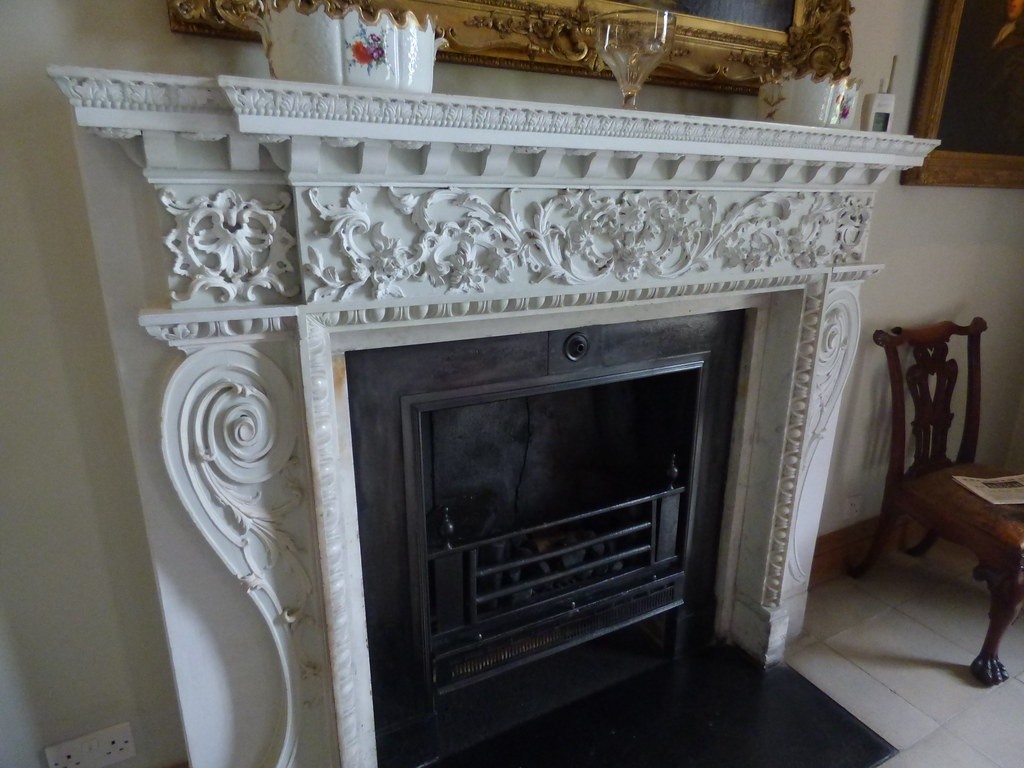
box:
[595,8,677,111]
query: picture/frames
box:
[168,0,855,98]
[899,0,1024,189]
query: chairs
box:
[849,316,1024,687]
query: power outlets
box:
[44,721,137,768]
